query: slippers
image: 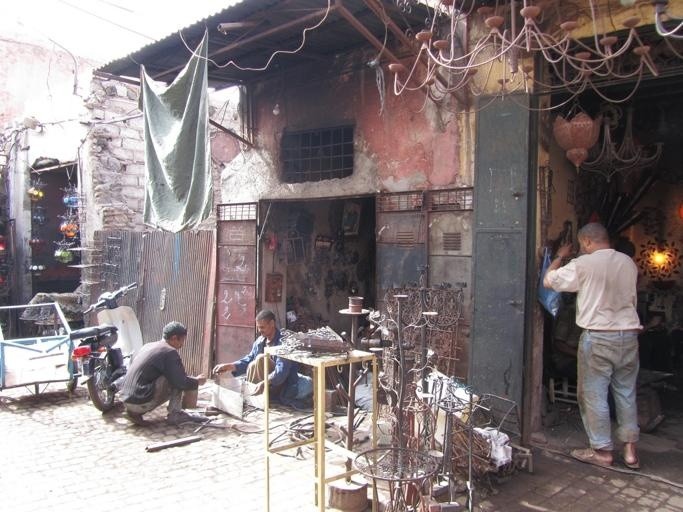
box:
[571,444,640,469]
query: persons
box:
[552,296,587,380]
[210,308,300,402]
[542,220,642,470]
[119,320,207,426]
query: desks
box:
[263,341,378,510]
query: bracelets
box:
[554,255,563,261]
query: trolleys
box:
[0,300,80,403]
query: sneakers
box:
[123,409,149,426]
[163,410,193,423]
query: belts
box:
[584,329,639,335]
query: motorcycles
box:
[69,282,143,412]
